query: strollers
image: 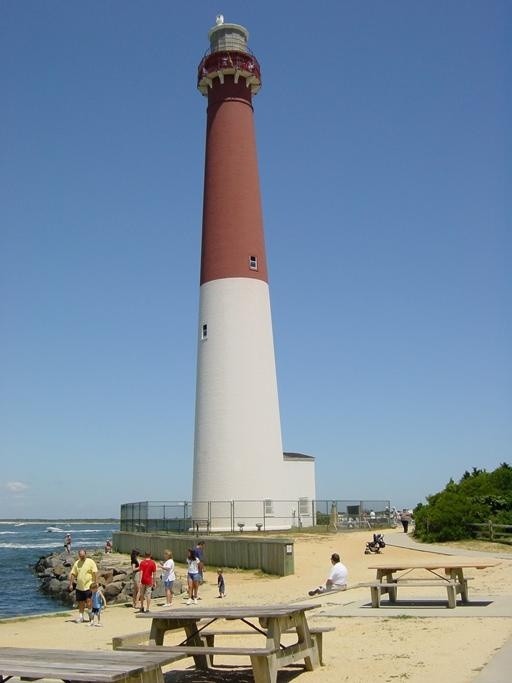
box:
[364,532,385,554]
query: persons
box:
[66,533,71,551]
[131,541,206,612]
[217,568,225,598]
[63,536,67,550]
[309,554,348,595]
[89,583,106,626]
[69,549,98,623]
[370,506,410,533]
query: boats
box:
[46,525,64,532]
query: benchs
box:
[111,625,338,683]
[358,576,476,609]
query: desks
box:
[366,554,503,609]
[0,645,188,683]
[135,602,323,683]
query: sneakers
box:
[187,597,198,605]
[309,588,320,596]
[76,618,102,627]
[163,603,172,607]
